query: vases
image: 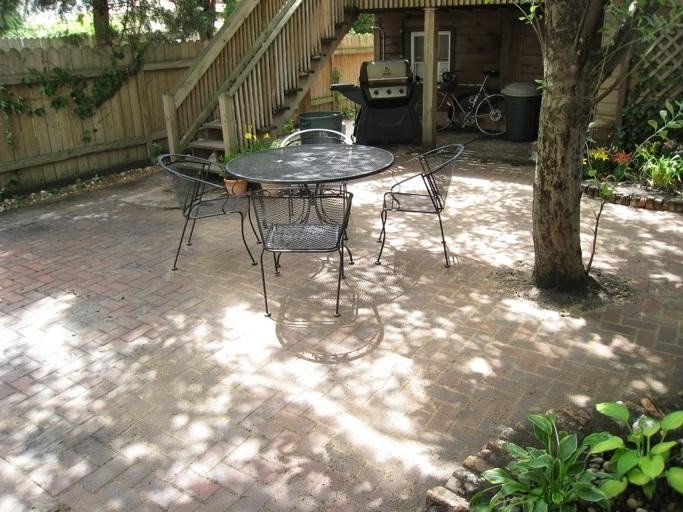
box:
[262,183,287,197]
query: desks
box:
[226,143,394,268]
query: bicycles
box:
[413,68,506,137]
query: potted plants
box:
[216,143,248,196]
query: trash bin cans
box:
[501,82,541,141]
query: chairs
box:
[158,153,262,272]
[279,129,358,217]
[258,185,353,318]
[375,142,466,268]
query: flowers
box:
[244,123,281,152]
